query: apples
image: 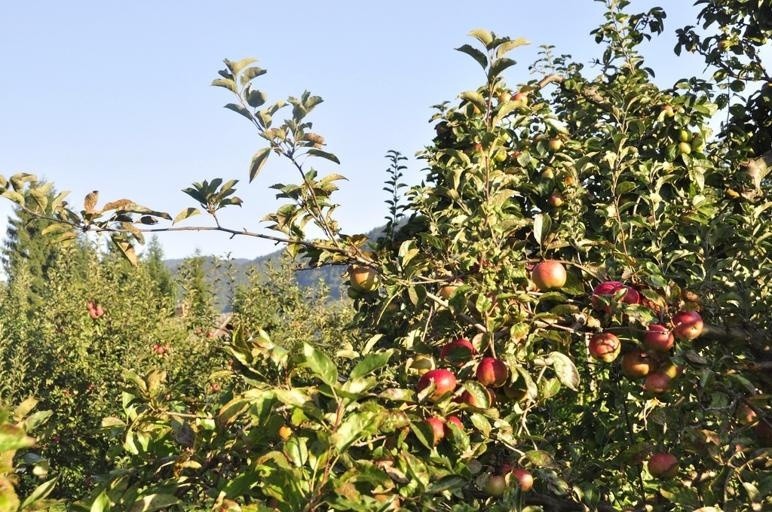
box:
[87,40,771,511]
